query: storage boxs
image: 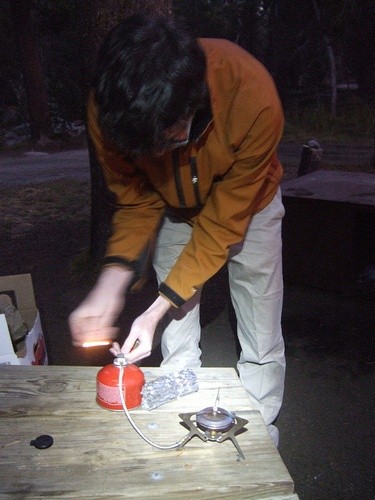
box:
[0,273,48,366]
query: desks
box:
[0,363,302,500]
[282,169,375,296]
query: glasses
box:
[124,111,189,153]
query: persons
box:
[68,23,286,448]
